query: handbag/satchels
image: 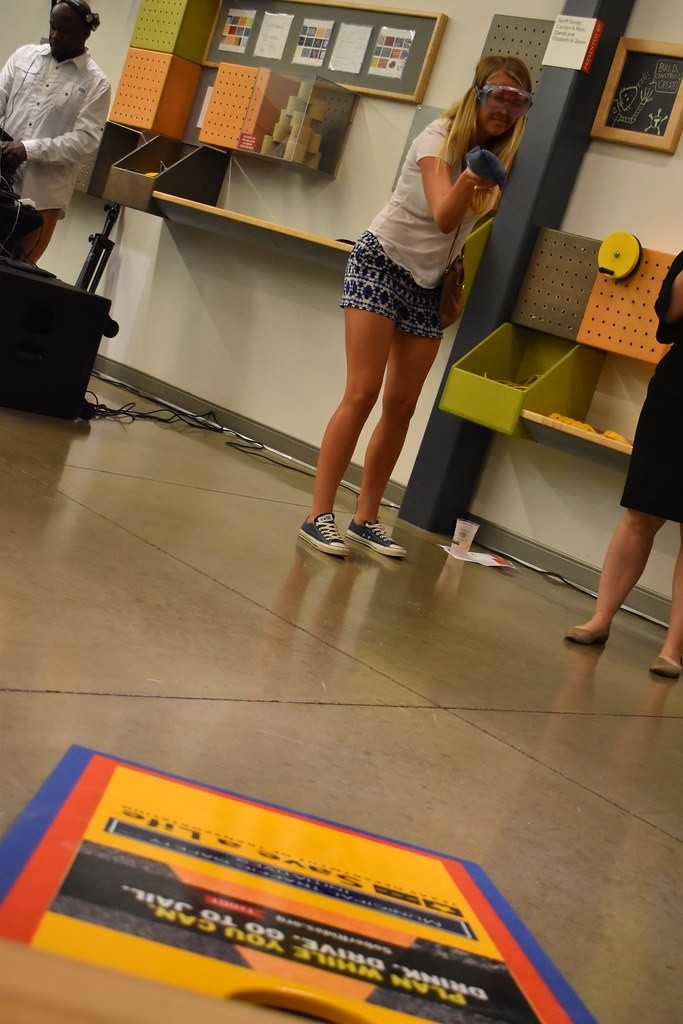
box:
[438,254,465,330]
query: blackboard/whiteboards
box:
[588,37,683,155]
[201,0,448,104]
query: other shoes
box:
[649,655,683,679]
[565,624,609,645]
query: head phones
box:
[51,0,100,31]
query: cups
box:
[449,519,480,556]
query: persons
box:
[296,55,535,557]
[564,250,683,680]
[0,0,110,267]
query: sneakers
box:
[298,513,349,555]
[346,512,407,556]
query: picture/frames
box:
[590,36,682,154]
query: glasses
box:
[476,85,534,116]
